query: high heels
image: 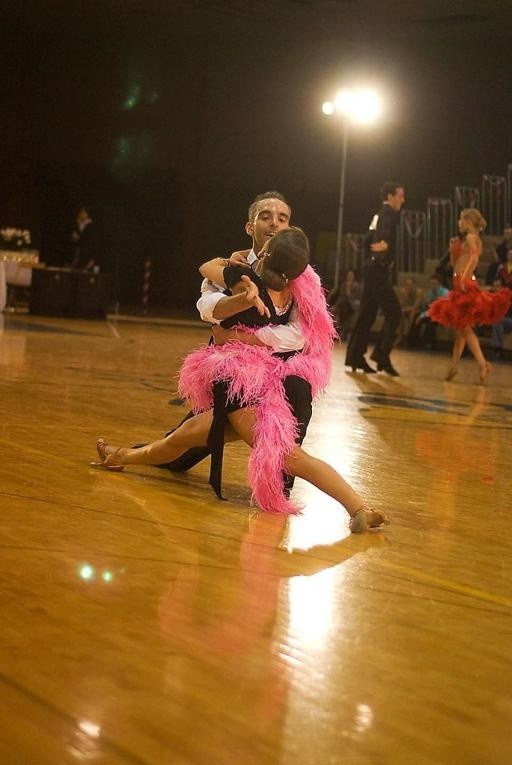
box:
[90,438,125,472]
[350,352,400,376]
[474,361,493,387]
[445,365,459,381]
[349,504,391,533]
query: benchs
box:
[354,226,512,357]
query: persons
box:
[328,270,361,342]
[69,205,97,270]
[326,225,512,349]
[97,227,387,533]
[345,182,405,377]
[429,209,512,384]
[127,192,312,500]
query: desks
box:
[31,268,109,320]
[1,251,38,329]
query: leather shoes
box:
[131,444,145,449]
[283,489,290,497]
